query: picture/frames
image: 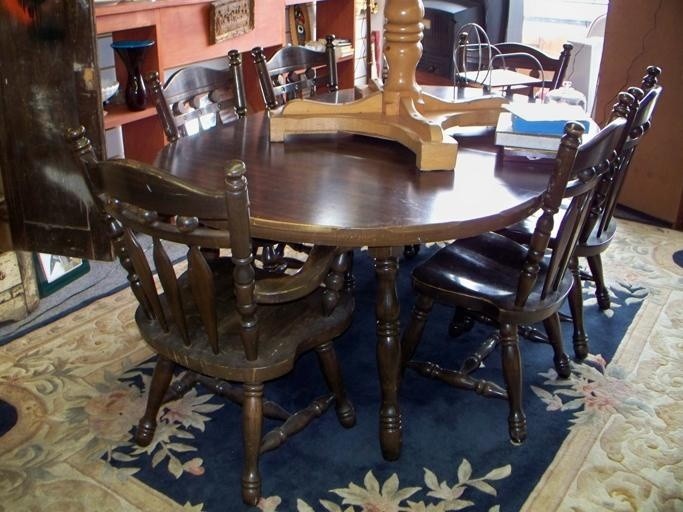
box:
[211,0,255,46]
[31,250,90,301]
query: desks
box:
[128,73,603,468]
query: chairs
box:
[495,63,661,365]
[141,49,312,276]
[249,32,339,110]
[456,32,574,95]
[64,121,364,508]
[402,91,636,444]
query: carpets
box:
[1,210,682,512]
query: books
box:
[494,96,601,160]
[310,37,355,59]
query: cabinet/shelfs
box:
[417,1,483,79]
